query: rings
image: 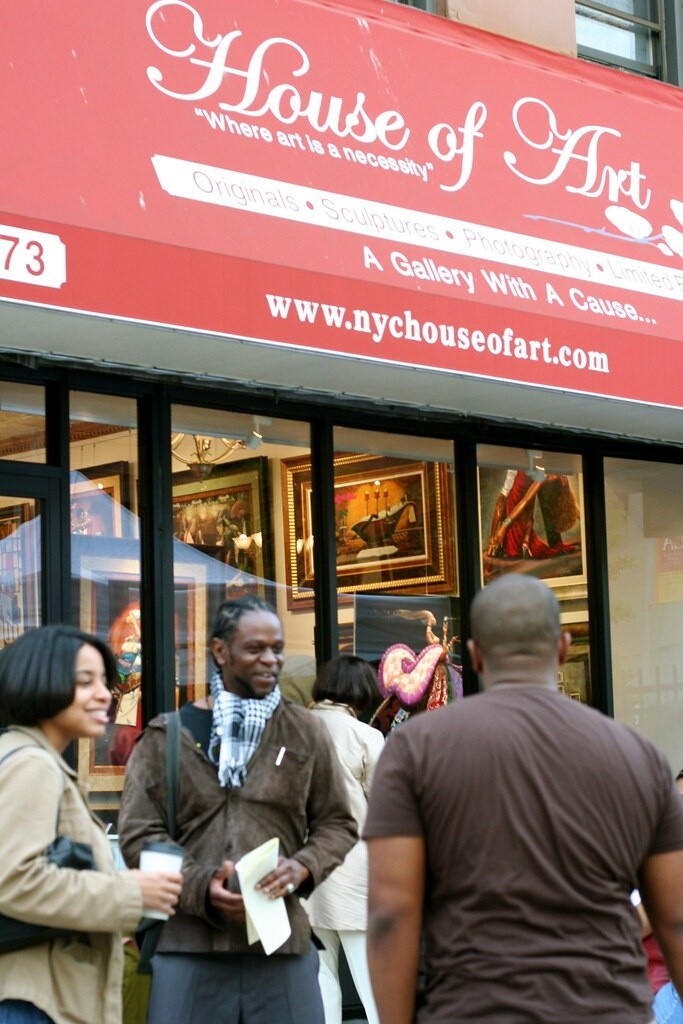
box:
[286,883,295,893]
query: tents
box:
[0,470,315,625]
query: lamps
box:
[170,432,243,483]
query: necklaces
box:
[206,696,213,712]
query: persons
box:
[115,598,359,1024]
[363,572,683,1024]
[299,655,386,1024]
[0,623,183,1024]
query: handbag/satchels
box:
[0,744,96,953]
[131,710,183,975]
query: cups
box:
[137,841,185,921]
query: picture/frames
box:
[280,452,451,613]
[476,463,590,601]
[1,503,37,648]
[66,459,129,640]
[63,533,225,803]
[558,620,596,710]
[167,455,275,612]
